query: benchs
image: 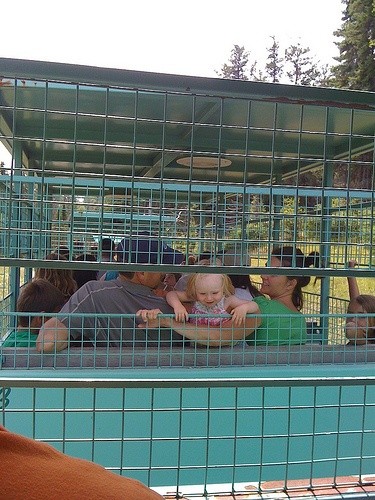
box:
[0,342,375,364]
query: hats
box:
[117,231,185,264]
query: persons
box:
[344,260,375,345]
[17,232,327,355]
